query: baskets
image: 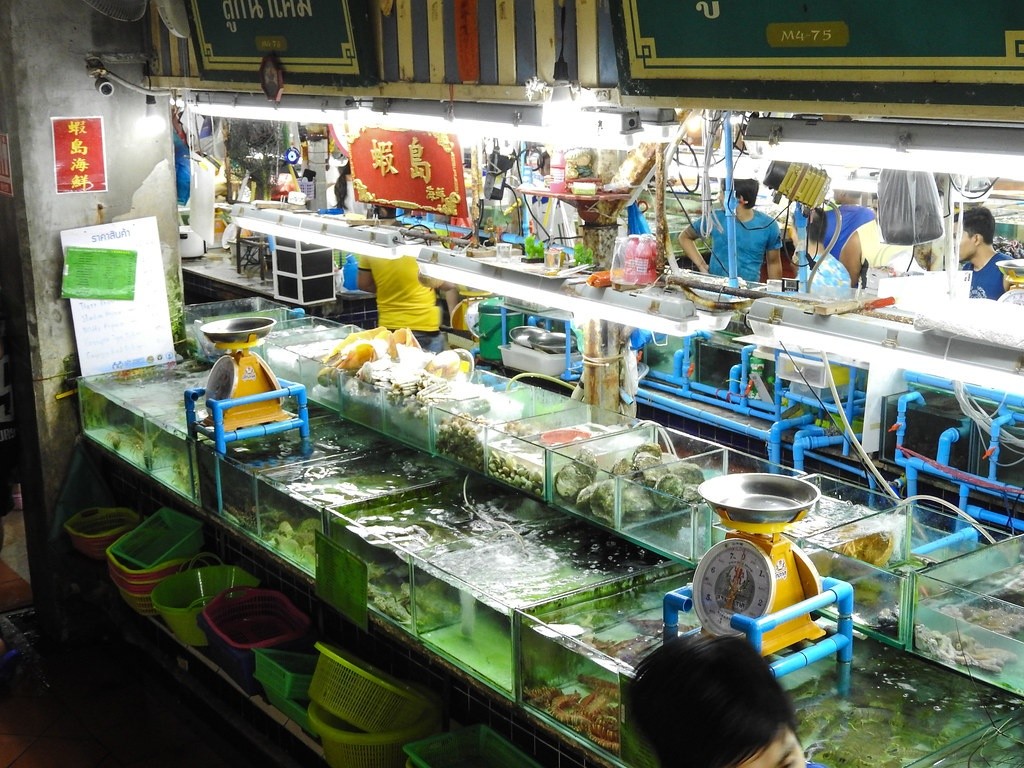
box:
[63,504,545,768]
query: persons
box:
[536,150,552,177]
[625,632,831,768]
[356,205,459,355]
[953,206,1016,302]
[677,178,783,284]
[326,159,389,219]
[790,206,851,295]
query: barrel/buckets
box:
[470,298,526,361]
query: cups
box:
[766,279,780,292]
[496,244,510,262]
[545,250,560,272]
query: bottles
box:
[623,233,656,285]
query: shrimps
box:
[780,650,979,768]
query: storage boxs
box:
[81,293,1024,768]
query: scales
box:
[222,223,250,250]
[692,473,826,656]
[199,316,289,431]
[452,289,493,342]
[995,259,1024,305]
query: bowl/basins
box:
[508,326,578,353]
